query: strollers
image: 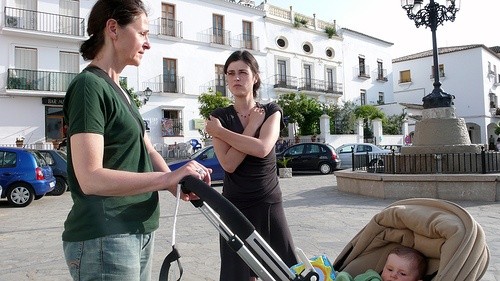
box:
[180,174,491,281]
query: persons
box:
[205,49,300,281]
[60,0,213,280]
[333,245,429,281]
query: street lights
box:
[401,0,461,109]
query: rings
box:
[197,169,203,174]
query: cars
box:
[378,144,404,154]
[335,143,393,170]
[37,150,68,196]
[276,143,341,174]
[0,146,57,207]
[167,145,225,184]
